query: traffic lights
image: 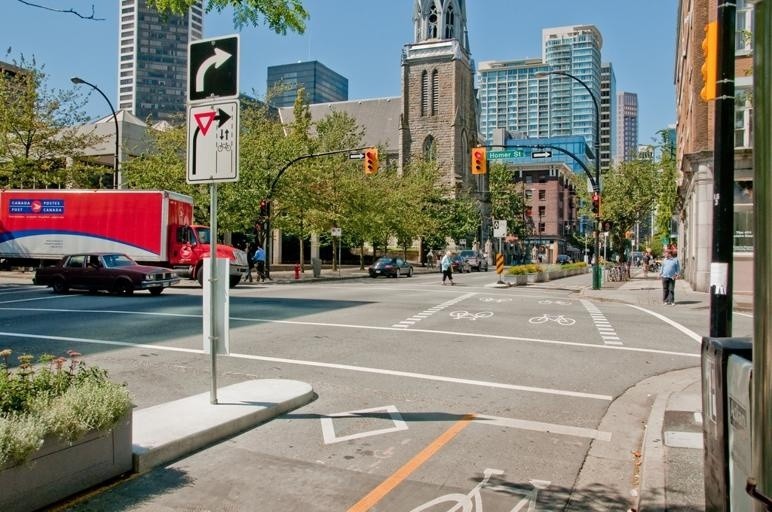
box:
[363,146,379,176]
[591,193,601,216]
[259,199,270,218]
[471,147,488,176]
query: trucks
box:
[1,189,250,292]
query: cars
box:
[440,253,468,275]
[368,255,415,280]
[31,249,182,299]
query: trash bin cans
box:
[701,336,755,512]
[592,266,602,288]
[313,257,322,277]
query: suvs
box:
[459,249,491,273]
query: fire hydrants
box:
[293,263,303,280]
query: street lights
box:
[533,69,605,292]
[68,75,123,189]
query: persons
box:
[440,251,456,286]
[251,244,266,282]
[635,252,659,279]
[426,249,434,270]
[244,245,254,282]
[657,251,681,306]
